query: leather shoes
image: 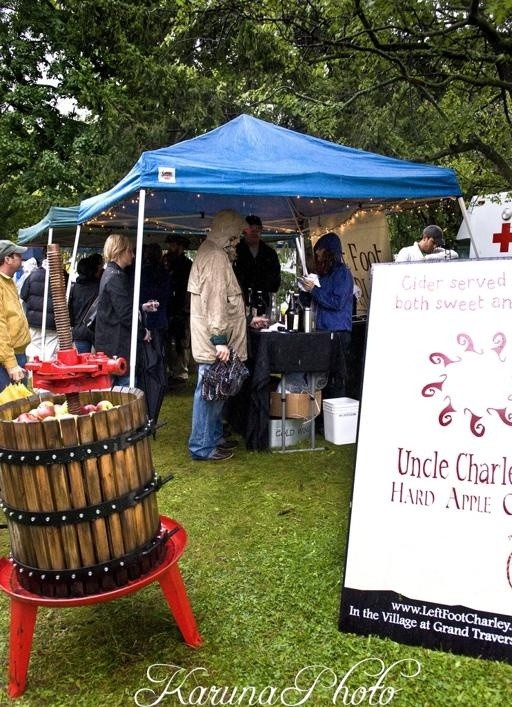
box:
[193,440,238,461]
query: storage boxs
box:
[267,416,313,450]
[267,386,322,418]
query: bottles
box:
[302,306,312,332]
[245,287,303,332]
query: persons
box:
[395,225,458,262]
[299,233,353,434]
[0,240,31,393]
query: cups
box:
[149,299,157,311]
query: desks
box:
[221,318,340,454]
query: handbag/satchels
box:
[83,296,100,331]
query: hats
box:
[245,216,262,224]
[423,225,445,246]
[0,240,28,258]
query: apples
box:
[14,400,118,422]
